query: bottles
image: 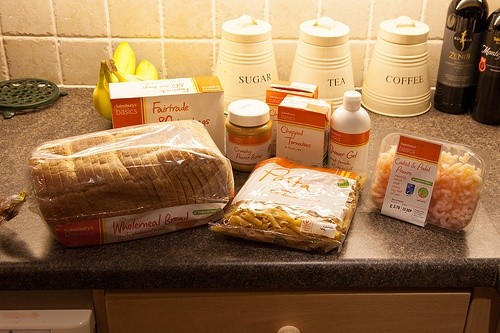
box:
[327,91,372,184]
[215,14,279,116]
[433,0,489,114]
[360,14,432,117]
[289,16,354,115]
[470,6,500,126]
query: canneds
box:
[224,99,272,172]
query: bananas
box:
[91,42,158,120]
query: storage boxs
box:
[109,77,224,157]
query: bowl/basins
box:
[360,127,491,240]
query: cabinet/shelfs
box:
[93,284,500,333]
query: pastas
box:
[208,176,364,251]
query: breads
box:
[27,120,234,236]
